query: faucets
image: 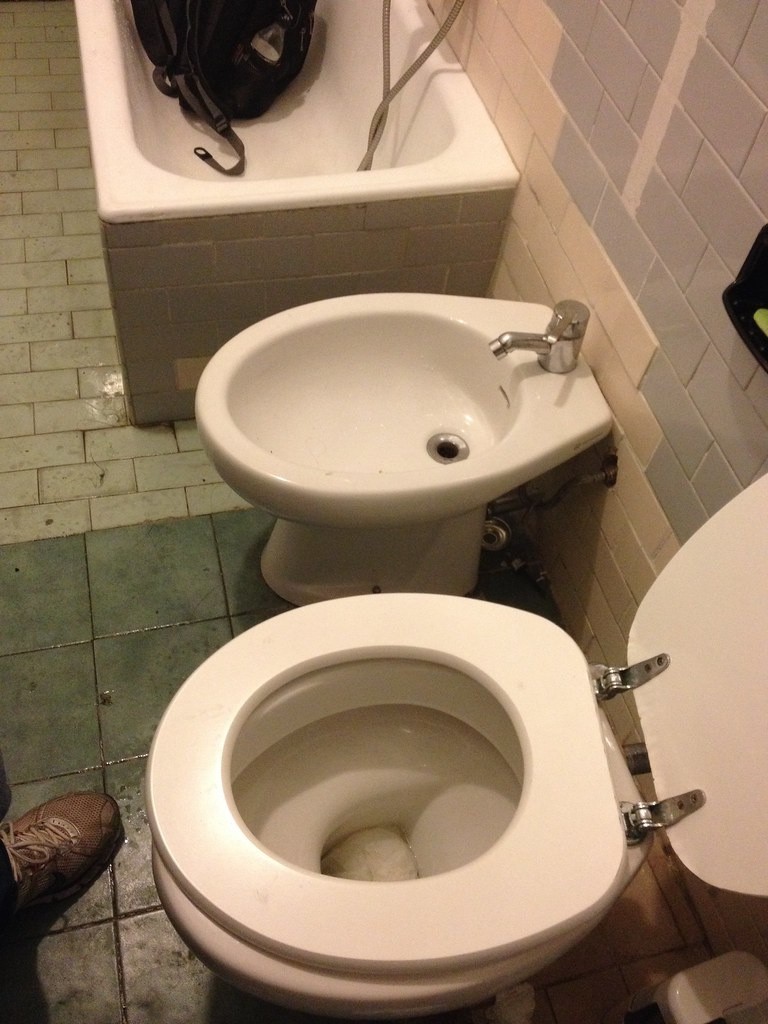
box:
[488,299,591,375]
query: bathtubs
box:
[74,0,523,431]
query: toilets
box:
[142,469,768,1022]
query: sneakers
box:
[0,791,122,915]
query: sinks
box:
[194,292,527,532]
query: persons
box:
[0,753,123,937]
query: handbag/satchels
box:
[131,0,317,137]
